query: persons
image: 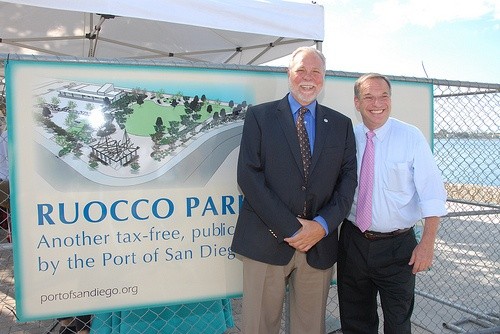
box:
[337,74,447,334]
[231,46,357,334]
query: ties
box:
[356,131,376,233]
[296,107,312,219]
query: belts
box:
[344,219,412,239]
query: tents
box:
[0,0,324,66]
